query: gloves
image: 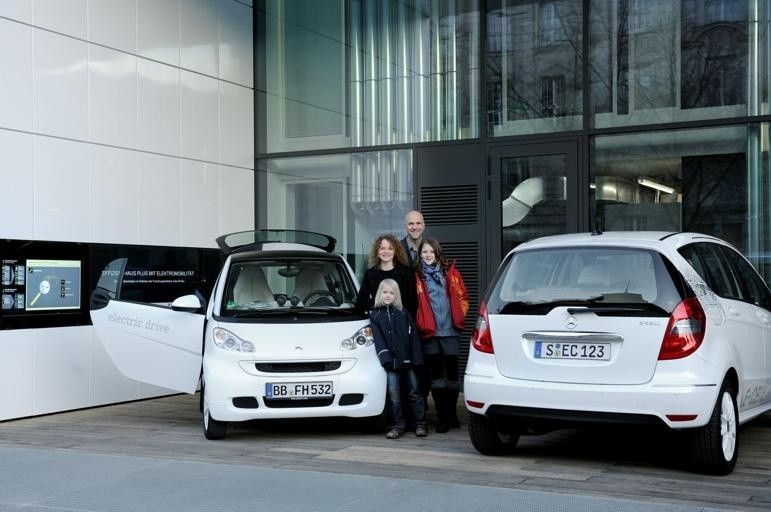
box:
[384,363,398,372]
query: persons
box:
[412,237,469,434]
[396,210,425,266]
[356,233,415,322]
[370,278,427,438]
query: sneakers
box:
[416,423,427,437]
[386,427,404,439]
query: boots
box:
[432,379,461,434]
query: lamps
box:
[638,175,676,194]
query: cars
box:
[463,204,771,474]
[89,229,387,439]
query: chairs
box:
[577,260,622,289]
[234,264,334,307]
